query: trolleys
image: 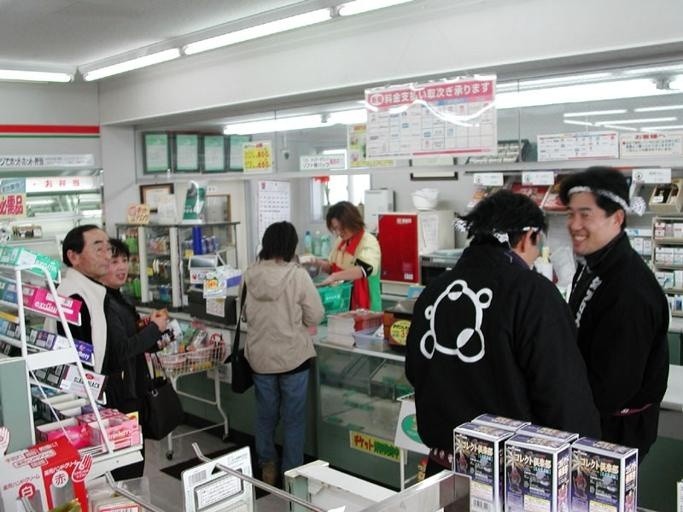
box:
[152,344,228,461]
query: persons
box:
[300,199,385,311]
[232,219,325,493]
[404,188,608,483]
[555,164,672,476]
[94,237,170,482]
[39,223,120,409]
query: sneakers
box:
[261,466,278,486]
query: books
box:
[320,305,384,349]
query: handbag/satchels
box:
[141,351,186,441]
[224,347,255,395]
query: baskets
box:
[314,277,355,325]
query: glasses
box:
[79,244,117,256]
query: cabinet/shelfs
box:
[0,149,683,512]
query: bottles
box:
[118,230,141,298]
[304,231,331,257]
